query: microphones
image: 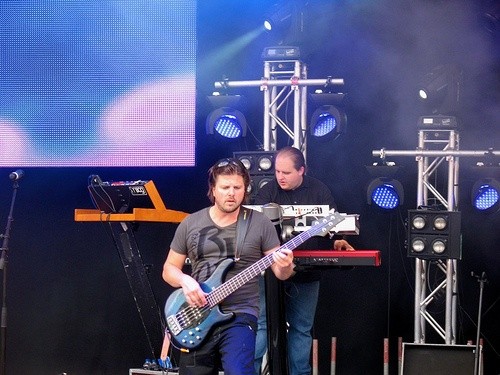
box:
[9,169,25,179]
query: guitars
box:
[163,208,346,353]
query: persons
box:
[162,159,294,375]
[253,147,355,375]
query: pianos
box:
[292,247,382,374]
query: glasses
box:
[216,160,242,168]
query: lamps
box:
[307,91,347,139]
[264,0,309,31]
[365,162,406,209]
[203,93,247,138]
[419,67,462,101]
[467,162,500,213]
